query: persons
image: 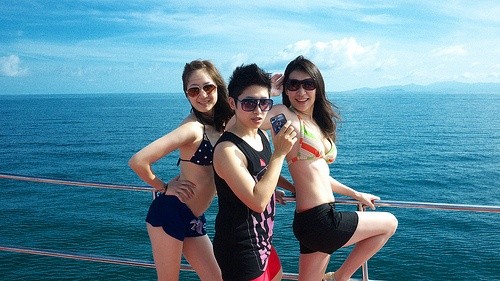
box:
[212,64,298,281]
[128,58,285,281]
[225,54,398,281]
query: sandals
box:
[322,271,335,281]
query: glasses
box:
[286,78,316,91]
[187,84,216,97]
[237,98,273,111]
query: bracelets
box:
[157,184,166,192]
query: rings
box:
[278,85,280,88]
[288,129,292,135]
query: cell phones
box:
[270,113,288,134]
